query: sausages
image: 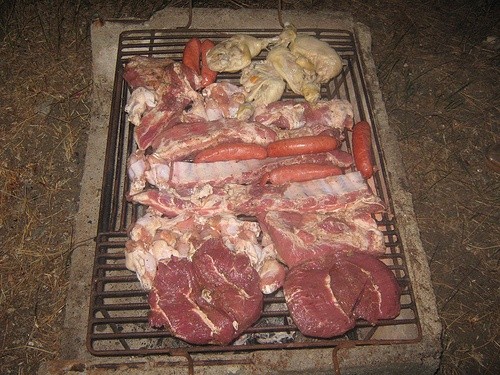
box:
[268,163,344,183]
[194,142,267,164]
[184,38,217,87]
[267,135,338,159]
[353,120,375,180]
